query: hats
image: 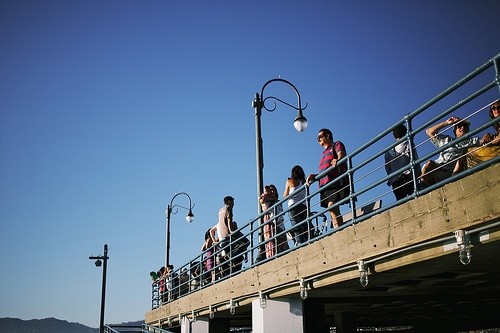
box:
[453,119,471,133]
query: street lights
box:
[88,243,110,333]
[165,192,196,276]
[250,78,309,266]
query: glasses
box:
[491,105,500,111]
[456,124,469,128]
[317,135,326,141]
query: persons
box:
[417,116,490,196]
[466,101,500,169]
[384,123,422,207]
[304,128,350,233]
[209,196,240,281]
[150,221,250,310]
[254,184,290,267]
[283,166,316,250]
